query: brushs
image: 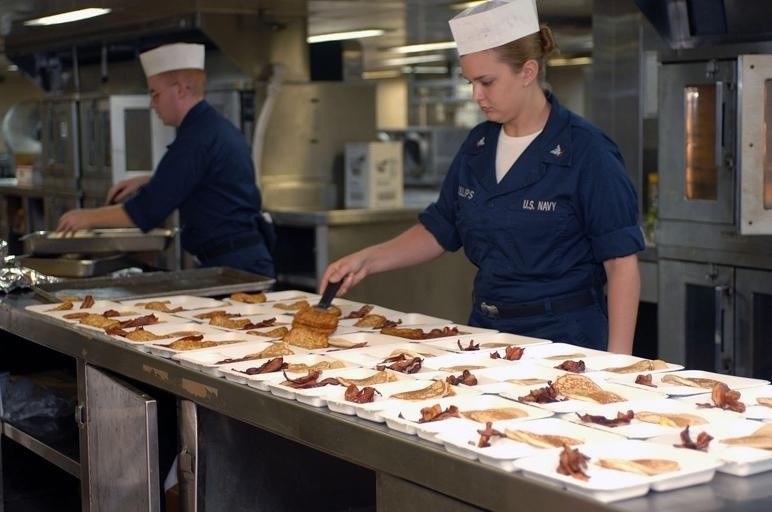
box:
[283,278,343,350]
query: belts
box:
[474,287,603,321]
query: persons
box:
[318,1,647,355]
[53,44,280,292]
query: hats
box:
[138,43,206,80]
[448,1,541,57]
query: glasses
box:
[148,80,178,101]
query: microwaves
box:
[368,126,468,191]
